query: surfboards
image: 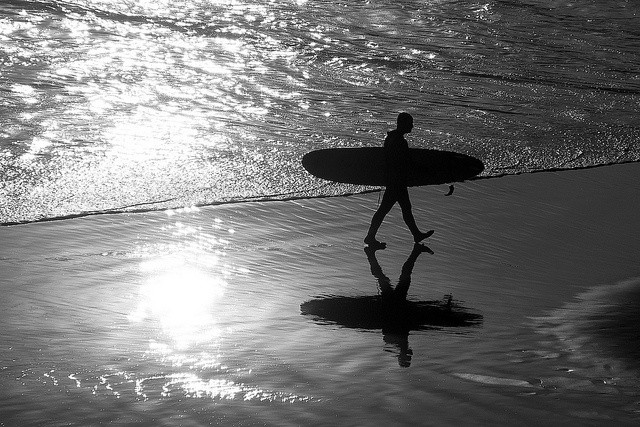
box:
[300,146,484,187]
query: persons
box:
[364,112,434,245]
